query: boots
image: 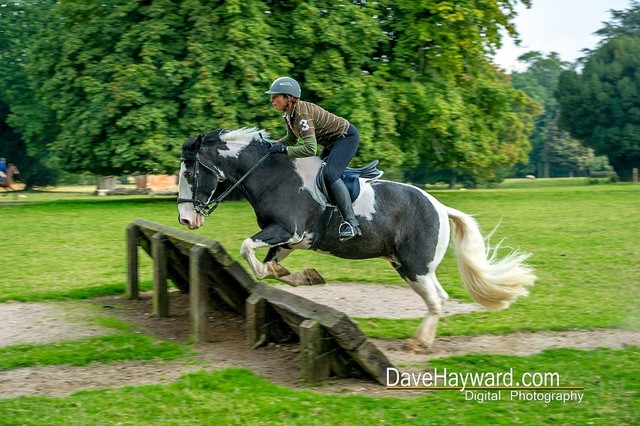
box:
[329,178,362,236]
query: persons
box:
[0,158,8,179]
[261,75,366,238]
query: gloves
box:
[269,143,288,154]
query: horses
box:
[176,126,538,356]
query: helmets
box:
[265,77,301,97]
